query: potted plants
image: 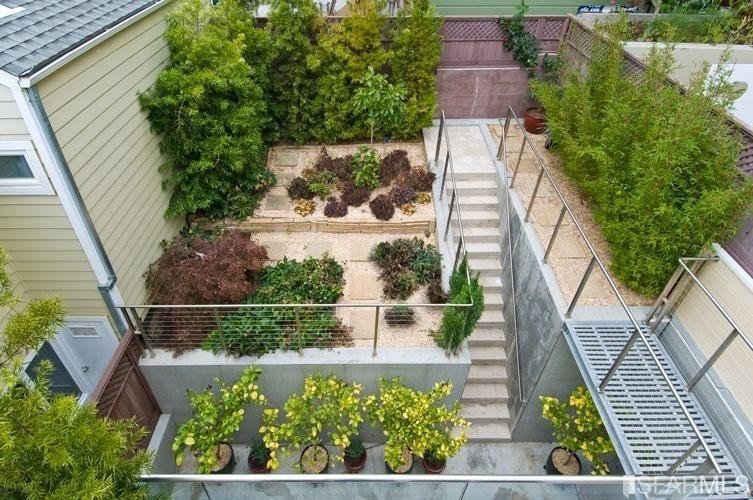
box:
[170,366,260,472]
[335,427,367,471]
[248,406,286,472]
[538,382,613,476]
[423,431,448,474]
[370,381,418,473]
[286,372,342,472]
[495,2,569,135]
